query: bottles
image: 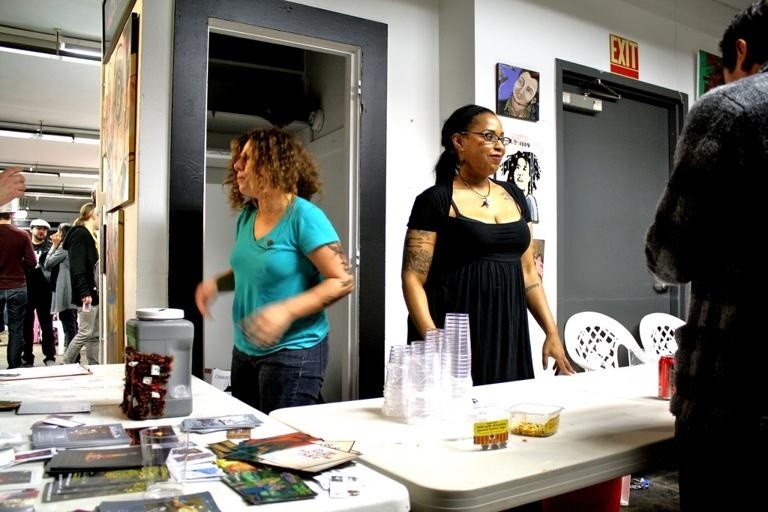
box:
[30,219,50,229]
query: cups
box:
[461,130,511,145]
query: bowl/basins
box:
[457,172,492,208]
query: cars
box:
[382,312,473,422]
[139,424,191,498]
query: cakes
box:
[658,355,679,400]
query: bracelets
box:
[508,404,563,438]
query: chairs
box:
[22,362,33,366]
[45,360,55,366]
[560,306,689,374]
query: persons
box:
[643,1,767,511]
[193,126,355,415]
[400,104,575,387]
[0,166,102,370]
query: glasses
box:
[0,23,103,69]
[0,158,102,200]
[1,120,76,145]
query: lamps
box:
[0,23,103,69]
[1,120,76,145]
[0,158,102,200]
[560,306,689,374]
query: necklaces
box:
[457,172,492,208]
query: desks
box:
[267,363,677,509]
[1,364,412,509]
